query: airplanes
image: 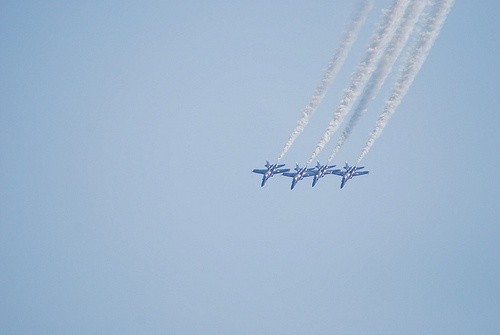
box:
[281,162,317,190]
[335,162,370,189]
[310,161,341,188]
[252,160,291,188]
